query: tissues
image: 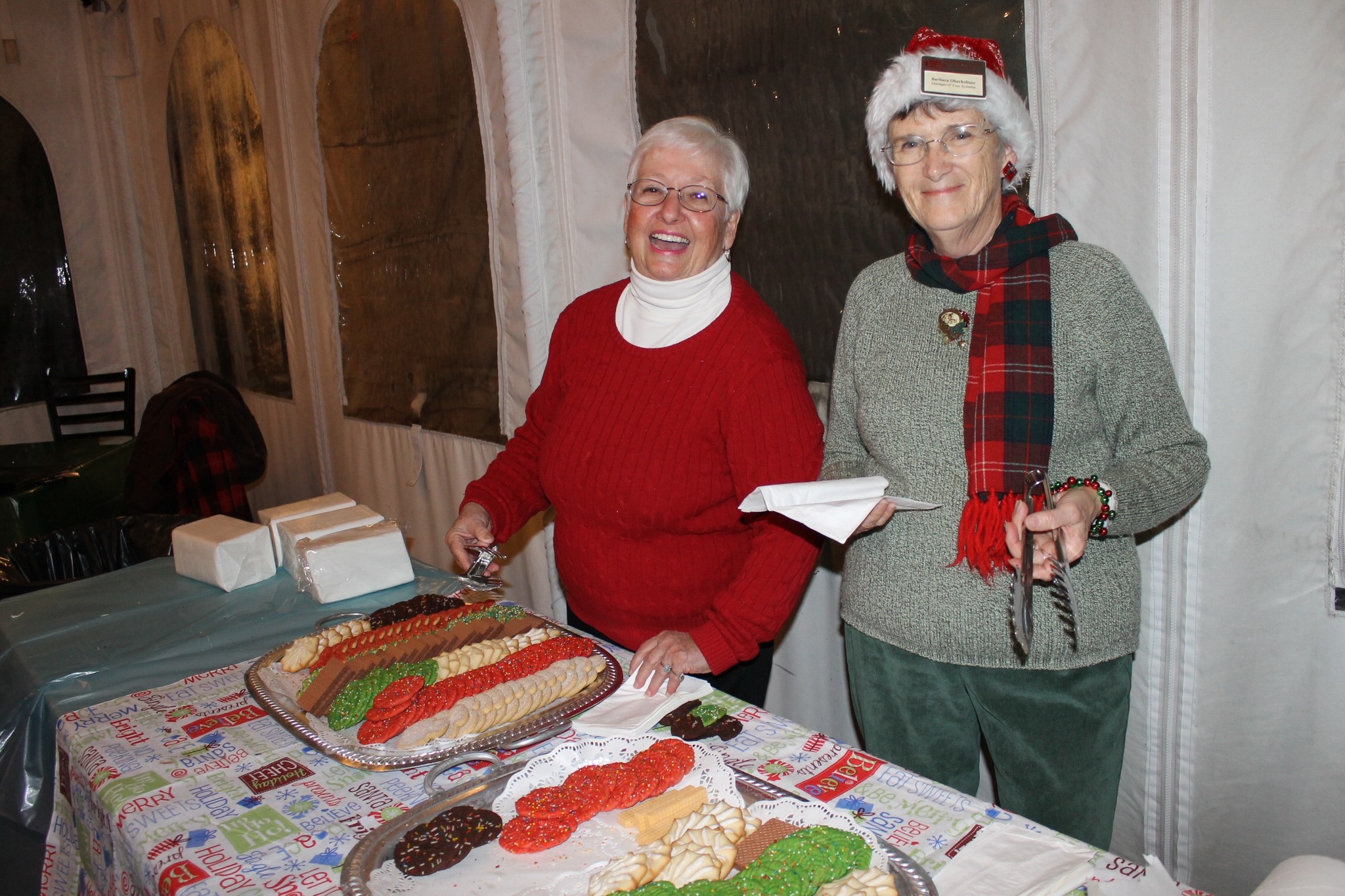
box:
[275,502,387,586]
[258,490,358,573]
[172,514,277,594]
[295,518,421,608]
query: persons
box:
[445,116,829,707]
[817,28,1210,858]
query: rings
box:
[671,669,685,682]
[660,661,672,673]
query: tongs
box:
[458,543,508,592]
[1008,467,1080,654]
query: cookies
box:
[273,589,614,746]
[396,701,902,895]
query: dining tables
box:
[2,533,1175,896]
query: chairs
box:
[40,367,140,570]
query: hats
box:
[866,26,1035,198]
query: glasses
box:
[883,122,1000,165]
[625,177,726,212]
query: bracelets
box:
[1050,475,1116,535]
